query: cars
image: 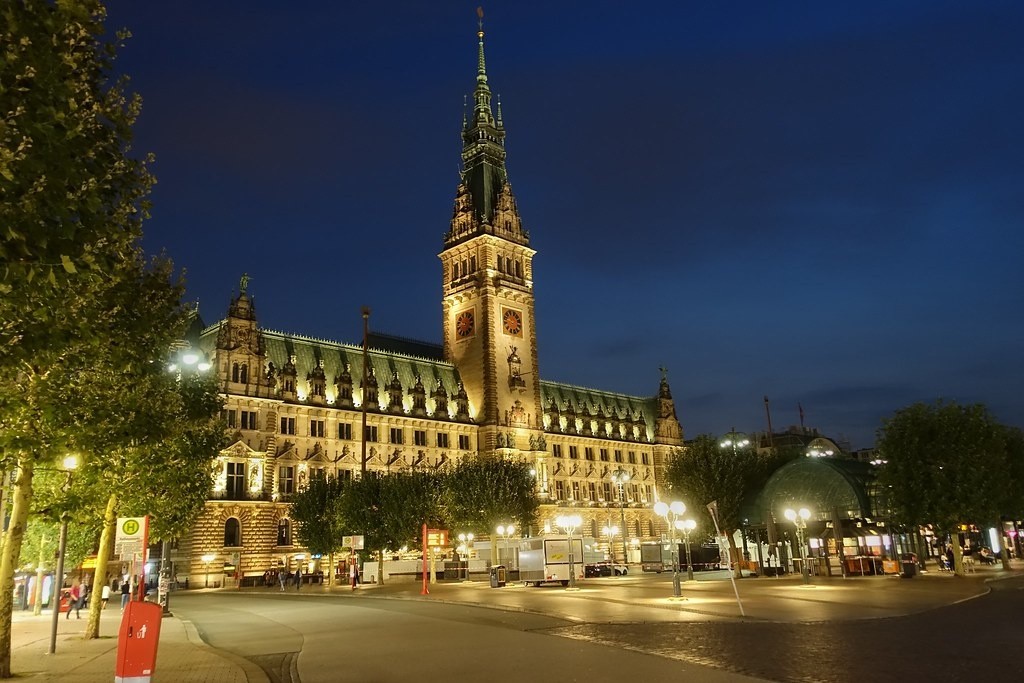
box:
[584,561,627,578]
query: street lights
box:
[48,455,81,654]
[675,520,698,582]
[458,532,474,584]
[556,515,580,591]
[497,526,515,586]
[602,526,619,579]
[202,555,214,590]
[782,509,816,588]
[654,502,688,601]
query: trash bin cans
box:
[902,552,917,577]
[115,601,161,683]
[490,565,506,588]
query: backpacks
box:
[280,572,285,580]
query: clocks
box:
[501,304,524,338]
[452,305,478,341]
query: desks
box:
[791,557,837,577]
[854,555,869,578]
[869,555,883,578]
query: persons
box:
[317,571,323,585]
[287,571,291,577]
[66,581,81,619]
[940,544,964,572]
[295,568,302,589]
[981,547,998,565]
[100,584,110,609]
[263,570,286,591]
[79,580,87,607]
[121,581,129,614]
[136,579,153,597]
[113,580,117,592]
[964,545,971,555]
